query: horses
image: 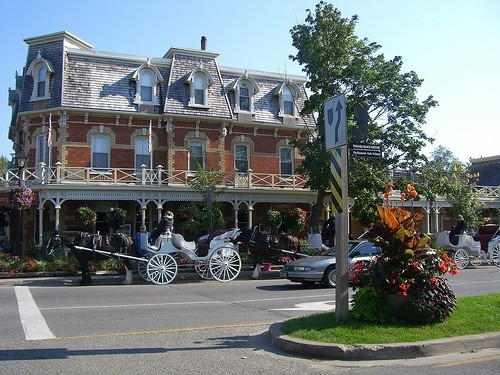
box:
[234,226,302,280]
[46,229,138,285]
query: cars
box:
[279,239,384,289]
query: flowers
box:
[6,184,35,210]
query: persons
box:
[449,215,465,239]
[148,211,174,245]
[135,225,151,253]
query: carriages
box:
[234,221,390,284]
[45,225,242,285]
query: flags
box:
[147,128,150,152]
[46,115,50,147]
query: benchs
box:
[153,231,171,249]
[198,228,241,257]
[458,233,482,249]
[172,235,196,250]
